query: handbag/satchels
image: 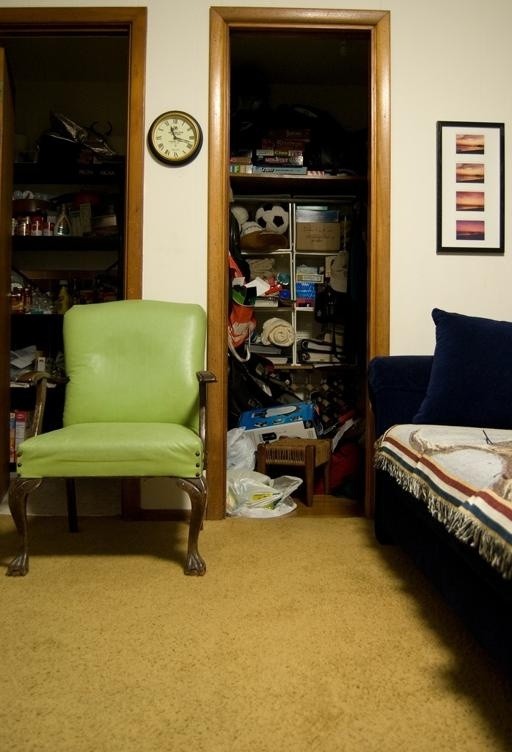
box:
[313,276,346,354]
[228,304,257,363]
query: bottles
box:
[52,205,71,236]
[23,280,71,315]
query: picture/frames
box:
[435,120,505,256]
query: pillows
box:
[413,306,512,431]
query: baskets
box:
[297,283,315,307]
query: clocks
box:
[146,109,205,167]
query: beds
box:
[4,297,219,577]
[367,350,512,690]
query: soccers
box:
[255,203,290,234]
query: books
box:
[230,126,312,176]
[242,329,344,366]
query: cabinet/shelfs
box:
[8,167,126,472]
[233,194,358,370]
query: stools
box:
[254,434,333,508]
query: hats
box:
[329,251,348,293]
